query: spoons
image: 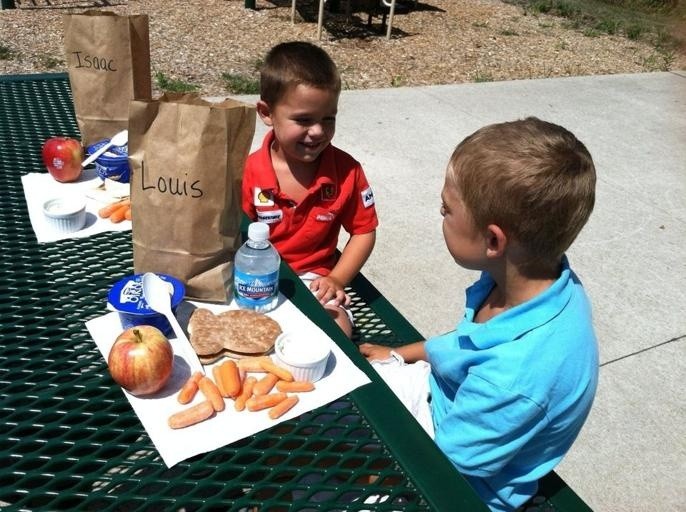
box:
[80,128,129,168]
[141,271,206,379]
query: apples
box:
[108,325,174,396]
[43,137,82,183]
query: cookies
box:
[188,308,282,364]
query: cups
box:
[41,195,88,235]
[273,330,331,384]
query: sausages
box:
[98,199,133,223]
[168,360,314,430]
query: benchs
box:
[345,255,599,512]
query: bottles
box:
[232,221,282,315]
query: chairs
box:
[290,0,396,42]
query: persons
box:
[247,115,602,510]
[242,40,378,340]
